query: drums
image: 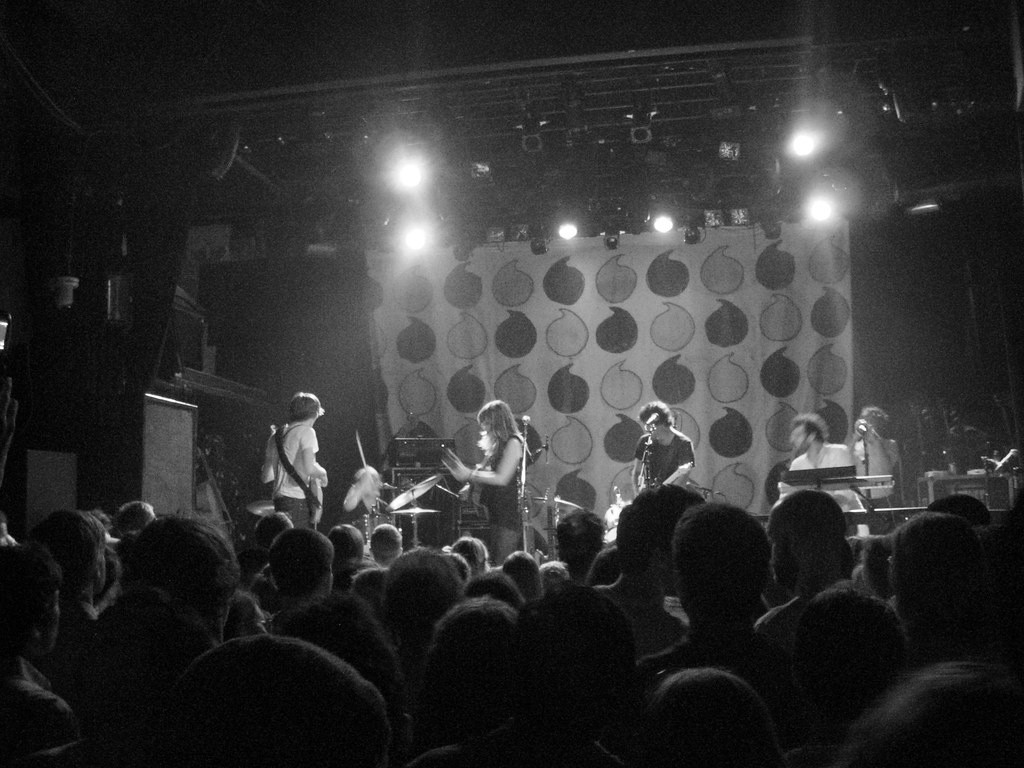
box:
[351,513,396,547]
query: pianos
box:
[779,474,896,495]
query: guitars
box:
[543,486,557,563]
[270,424,323,522]
[438,443,493,529]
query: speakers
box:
[459,527,492,554]
[18,393,200,539]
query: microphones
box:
[685,482,698,488]
[383,483,398,490]
[646,412,660,426]
[995,448,1022,471]
[545,434,549,464]
[858,425,867,434]
[522,415,530,422]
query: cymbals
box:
[532,496,583,512]
[390,474,443,509]
[390,506,442,517]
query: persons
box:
[342,464,397,549]
[441,399,528,567]
[850,404,901,523]
[260,390,330,534]
[0,484,1024,768]
[780,412,859,514]
[629,400,698,496]
